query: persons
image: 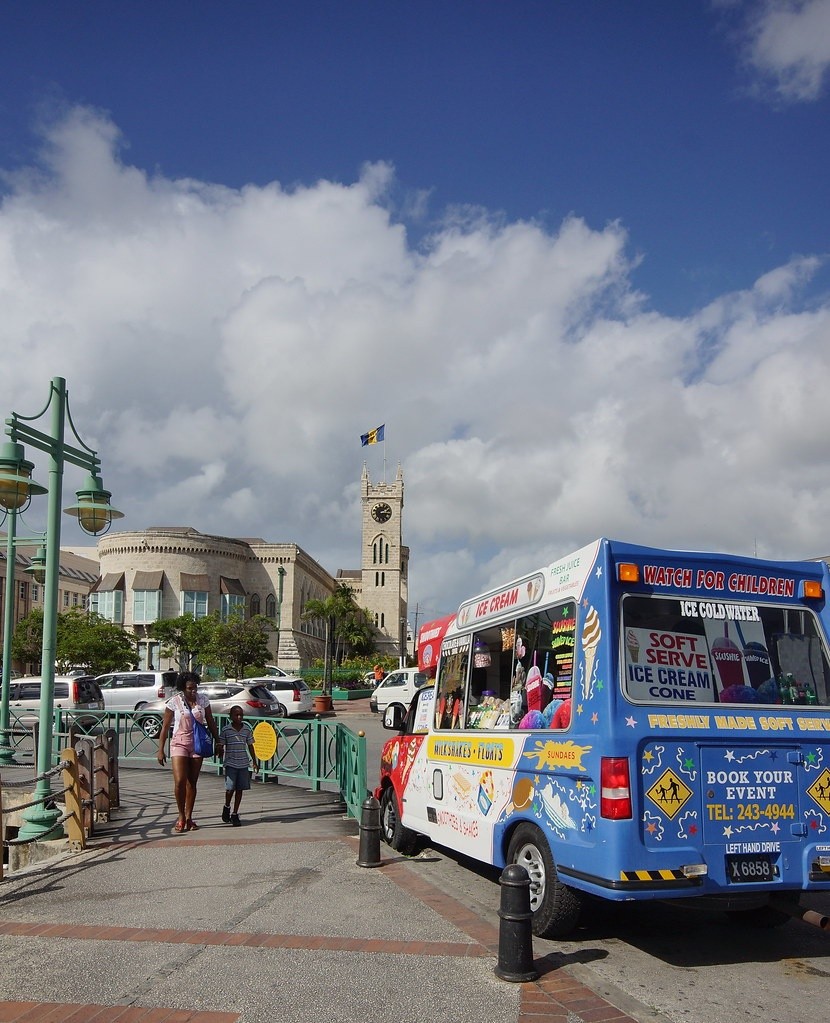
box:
[374,662,384,687]
[158,672,224,833]
[132,664,156,671]
[218,706,260,827]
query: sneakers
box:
[221,805,242,827]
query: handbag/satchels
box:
[183,698,213,757]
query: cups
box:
[524,665,542,712]
[743,641,770,691]
[711,637,745,689]
[541,673,554,713]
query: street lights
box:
[0,506,47,764]
[0,377,124,841]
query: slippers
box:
[175,818,199,833]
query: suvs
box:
[133,681,284,739]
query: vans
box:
[370,666,429,713]
[243,676,312,717]
[95,671,178,717]
[0,676,105,737]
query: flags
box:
[359,424,384,447]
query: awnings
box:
[219,575,247,596]
[131,569,164,591]
[88,571,125,597]
[179,572,210,592]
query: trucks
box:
[374,538,830,939]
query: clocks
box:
[371,502,392,524]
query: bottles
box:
[805,682,815,704]
[796,679,805,698]
[787,672,800,704]
[805,689,810,698]
[777,674,788,705]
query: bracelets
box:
[215,743,224,746]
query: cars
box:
[363,671,396,686]
